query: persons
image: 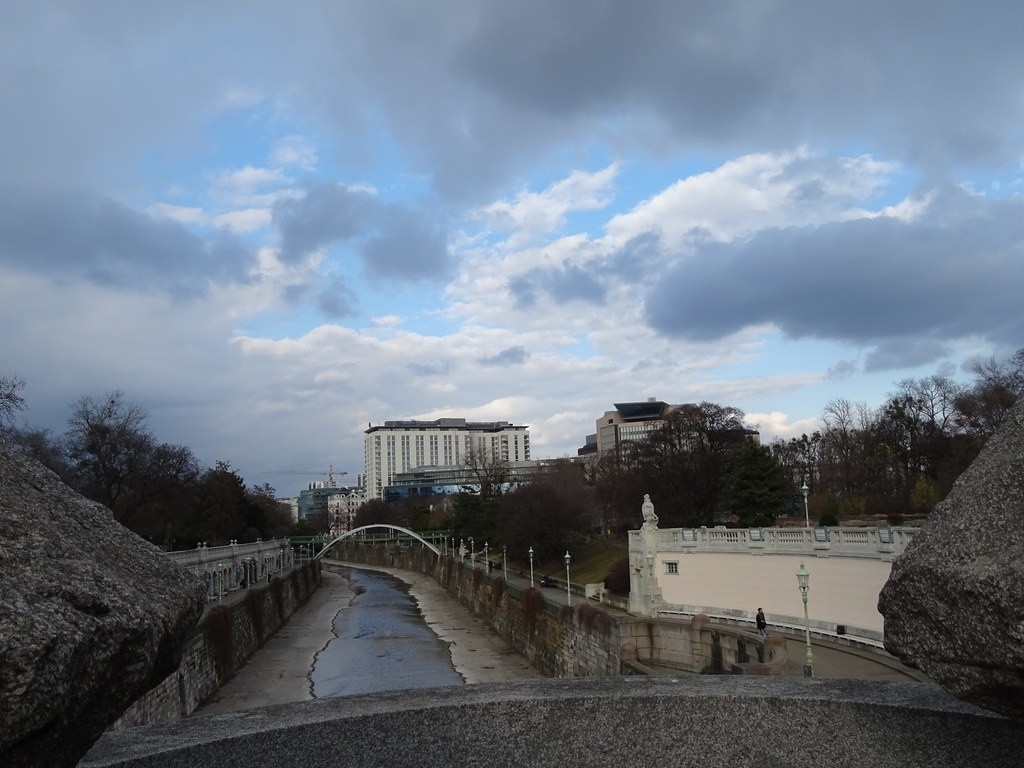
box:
[268,572,271,583]
[541,573,549,587]
[489,560,493,574]
[240,579,246,589]
[756,608,768,635]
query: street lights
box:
[564,550,571,606]
[246,555,250,589]
[217,561,223,601]
[291,547,294,567]
[801,481,809,529]
[503,544,507,581]
[646,550,656,618]
[529,547,534,588]
[280,549,283,574]
[265,552,269,579]
[484,541,489,573]
[299,538,315,565]
[795,562,815,678]
[432,533,464,563]
[471,540,474,568]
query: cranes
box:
[257,464,348,480]
